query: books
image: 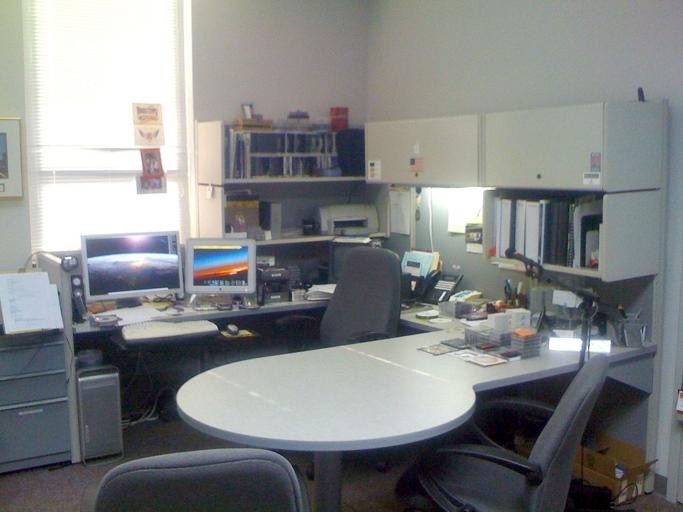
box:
[399,250,440,277]
[439,337,471,350]
[465,354,508,367]
[491,192,600,268]
[229,131,336,178]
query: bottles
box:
[330,107,349,132]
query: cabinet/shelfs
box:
[0,329,71,473]
[196,103,668,282]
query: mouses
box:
[226,322,239,335]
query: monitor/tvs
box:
[81,231,184,311]
[185,238,257,310]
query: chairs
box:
[92,448,309,509]
[394,353,609,512]
[263,247,401,355]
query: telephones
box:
[416,271,463,305]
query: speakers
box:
[72,274,87,315]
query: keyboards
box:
[122,320,218,344]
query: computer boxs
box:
[76,356,125,468]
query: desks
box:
[72,288,657,512]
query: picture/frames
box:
[0,117,23,199]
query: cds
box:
[95,316,116,327]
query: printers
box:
[317,203,379,236]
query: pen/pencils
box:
[503,280,523,298]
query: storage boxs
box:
[573,431,659,506]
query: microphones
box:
[505,248,542,269]
